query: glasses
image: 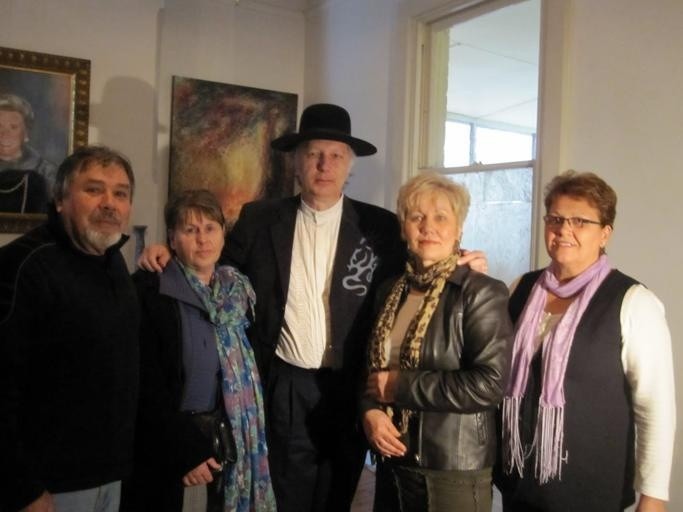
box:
[544,215,605,227]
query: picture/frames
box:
[0,46,91,235]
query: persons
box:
[1,144,143,512]
[0,93,59,214]
[360,173,511,512]
[137,104,487,512]
[128,189,247,512]
[492,173,678,512]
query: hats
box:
[270,103,377,156]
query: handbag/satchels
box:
[184,368,237,466]
